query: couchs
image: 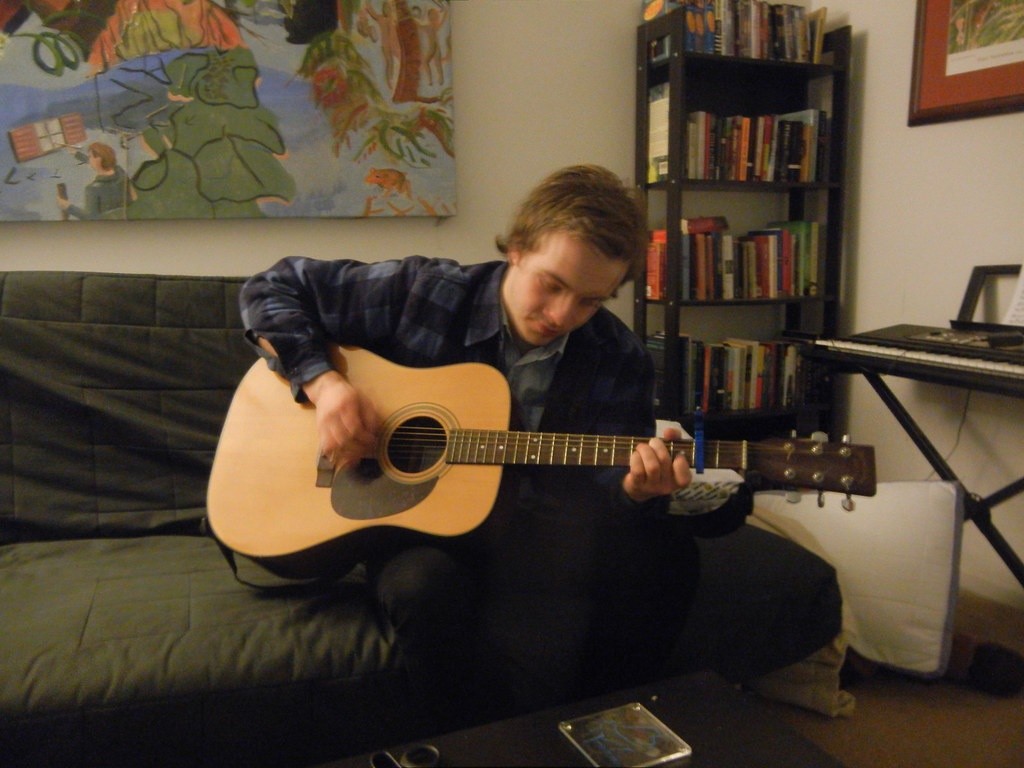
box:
[1,268,839,768]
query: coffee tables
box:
[326,669,852,766]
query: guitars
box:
[203,340,877,594]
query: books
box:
[645,331,821,415]
[641,0,810,62]
[645,81,827,185]
[643,216,827,300]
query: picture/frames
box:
[909,0,1024,125]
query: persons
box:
[237,164,701,715]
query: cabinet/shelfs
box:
[635,7,852,441]
